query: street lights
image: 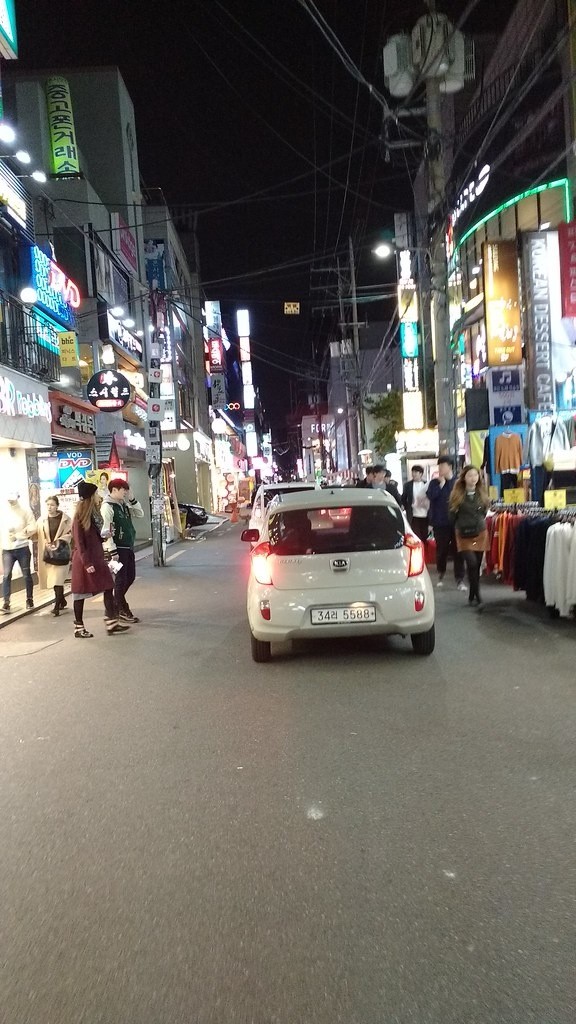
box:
[372,244,456,456]
[306,437,327,487]
[315,405,344,488]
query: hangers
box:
[491,499,576,525]
[503,423,512,434]
[535,411,576,419]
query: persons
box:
[401,466,431,541]
[71,482,130,638]
[0,489,73,617]
[100,479,144,623]
[448,465,491,611]
[425,456,467,591]
[355,465,401,506]
[98,473,109,499]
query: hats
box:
[5,491,21,501]
[78,482,99,500]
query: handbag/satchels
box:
[43,539,70,566]
[459,524,480,538]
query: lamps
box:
[0,150,31,163]
[0,123,16,143]
[17,170,47,183]
[21,287,37,309]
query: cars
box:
[246,483,324,551]
[240,487,436,662]
[150,496,208,529]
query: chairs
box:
[282,514,312,554]
[350,510,389,547]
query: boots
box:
[74,621,93,638]
[104,617,129,634]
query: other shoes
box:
[119,612,138,624]
[0,604,10,614]
[468,599,475,608]
[456,583,468,591]
[26,599,34,610]
[51,609,59,617]
[479,603,488,613]
[437,581,443,588]
[59,600,67,609]
